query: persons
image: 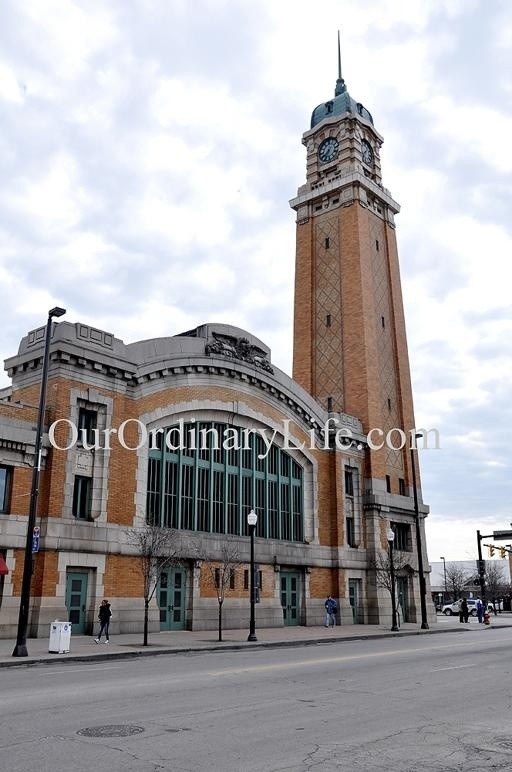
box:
[475,597,483,623]
[325,595,337,628]
[461,598,470,623]
[458,600,464,623]
[94,600,112,644]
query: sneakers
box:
[94,639,100,644]
[104,640,108,643]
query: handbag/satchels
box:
[477,603,481,609]
[333,607,337,613]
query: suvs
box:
[442,599,483,617]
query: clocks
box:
[318,137,373,165]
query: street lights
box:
[407,431,429,629]
[386,528,399,632]
[9,306,67,657]
[247,509,258,641]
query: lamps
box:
[194,553,205,568]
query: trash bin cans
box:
[48,620,72,654]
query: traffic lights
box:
[502,548,506,558]
[490,545,495,556]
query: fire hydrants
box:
[484,613,491,625]
